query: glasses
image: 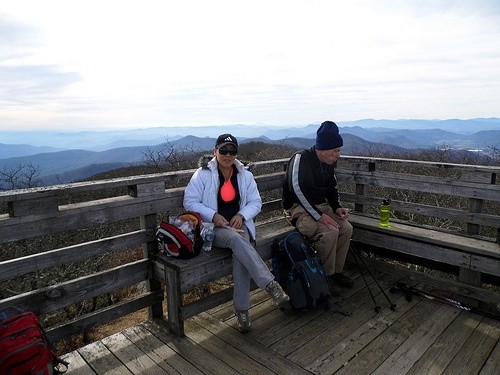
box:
[219,148,237,156]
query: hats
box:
[215,134,239,149]
[315,121,343,149]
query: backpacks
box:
[271,231,334,312]
[0,306,70,375]
[155,211,205,259]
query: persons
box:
[283,121,354,295]
[183,134,290,332]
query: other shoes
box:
[328,273,353,288]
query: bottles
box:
[380,199,390,227]
[203,228,214,251]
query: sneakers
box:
[235,308,252,333]
[265,280,290,306]
